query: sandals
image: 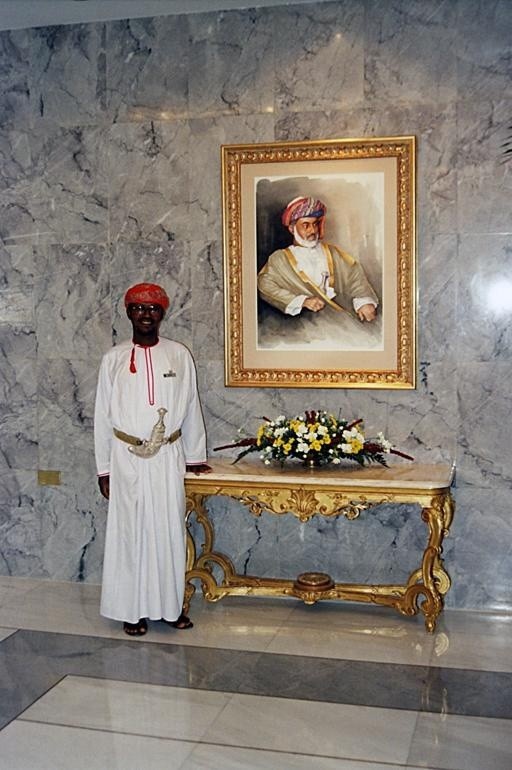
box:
[122,620,147,635]
[161,613,191,630]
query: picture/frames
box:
[220,136,416,391]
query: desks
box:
[179,457,459,634]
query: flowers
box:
[214,406,414,470]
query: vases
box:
[291,453,331,468]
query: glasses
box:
[128,302,162,316]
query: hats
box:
[282,196,328,240]
[124,282,170,307]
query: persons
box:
[93,282,214,637]
[258,194,384,351]
